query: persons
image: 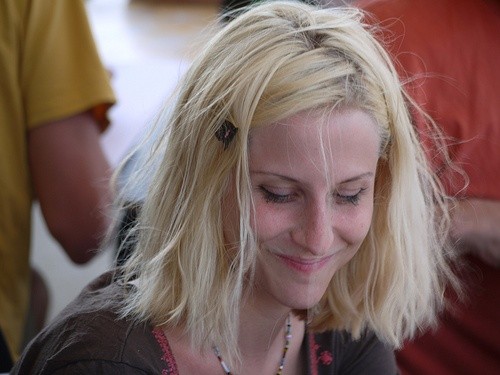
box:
[6,0,471,375]
[0,0,116,374]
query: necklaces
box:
[199,305,292,374]
